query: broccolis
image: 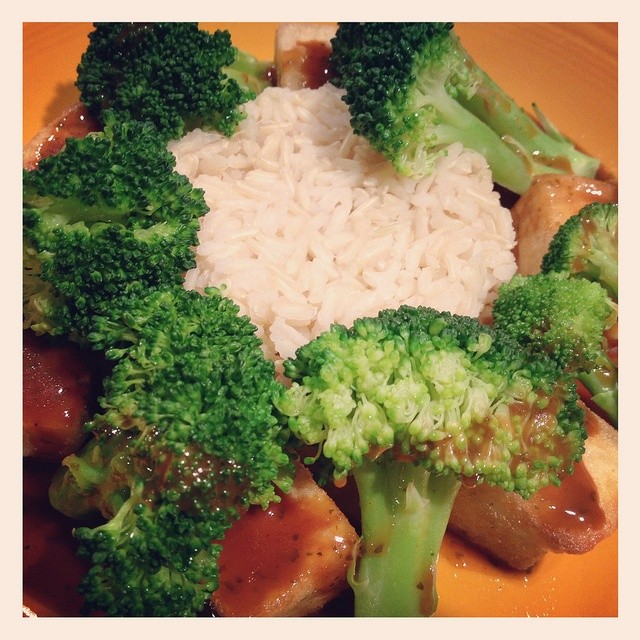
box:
[282,304,587,617]
[490,270,617,425]
[22,118,212,353]
[47,287,296,618]
[74,22,276,139]
[326,22,570,197]
[540,204,618,303]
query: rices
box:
[164,81,520,390]
